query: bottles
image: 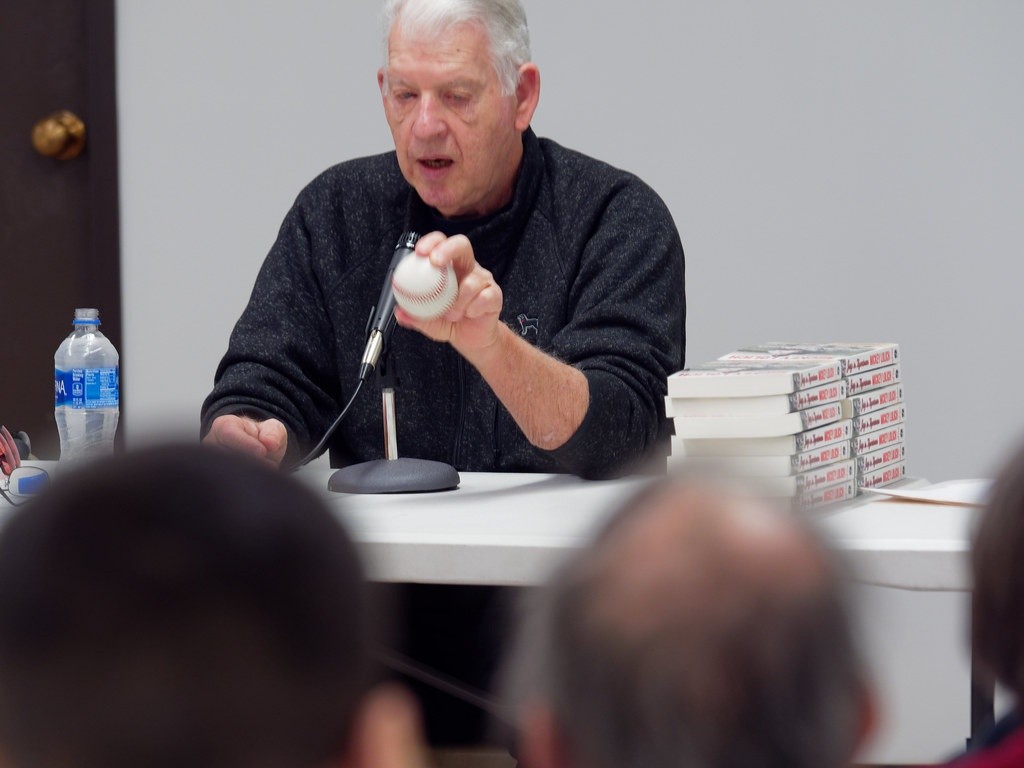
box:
[54,307,120,469]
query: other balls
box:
[392,251,457,321]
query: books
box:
[664,341,907,514]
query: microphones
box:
[359,231,420,383]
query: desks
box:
[1,458,1018,746]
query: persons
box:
[0,443,1024,768]
[199,0,687,480]
